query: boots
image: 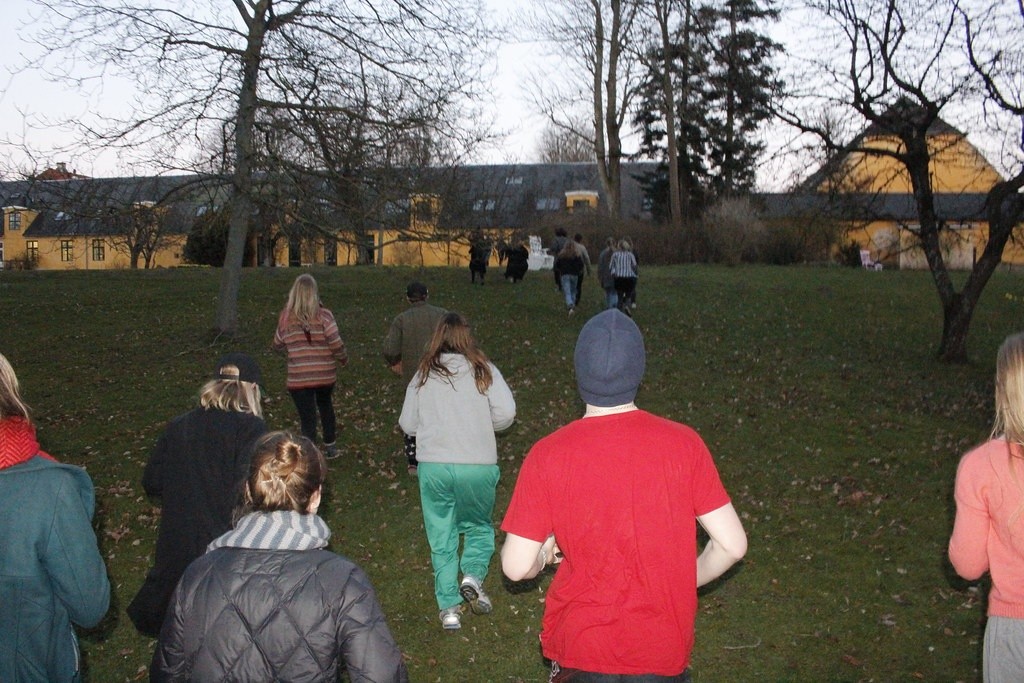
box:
[325,442,341,459]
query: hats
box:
[572,309,647,408]
[213,351,264,387]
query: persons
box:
[398,313,515,628]
[467,225,529,285]
[126,351,270,638]
[546,228,591,308]
[0,350,111,683]
[274,274,346,459]
[500,309,749,683]
[555,241,584,321]
[599,236,639,310]
[384,283,450,466]
[609,240,638,311]
[948,332,1024,683]
[150,431,409,683]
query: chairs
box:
[859,249,882,272]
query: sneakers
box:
[624,306,631,315]
[408,465,418,476]
[441,612,462,629]
[632,304,636,309]
[460,575,493,615]
[567,310,575,320]
